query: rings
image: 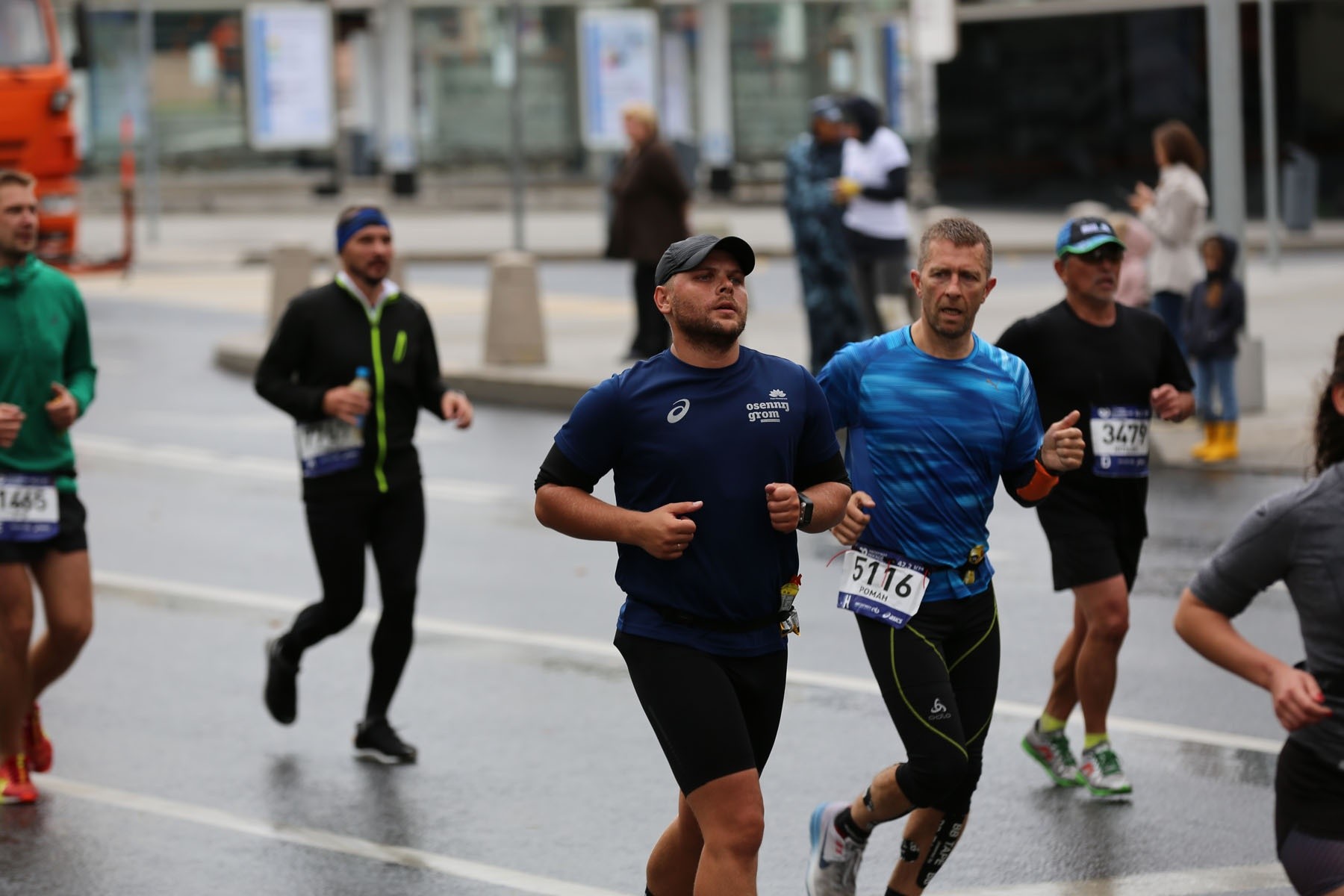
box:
[675,543,680,552]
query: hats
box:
[654,233,755,302]
[807,96,844,121]
[336,207,391,254]
[1056,215,1126,259]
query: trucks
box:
[0,0,83,276]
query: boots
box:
[1189,417,1237,463]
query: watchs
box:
[797,491,813,529]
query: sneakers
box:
[352,719,418,764]
[803,799,862,896]
[1076,739,1133,800]
[262,633,301,724]
[1021,716,1087,789]
[0,752,40,805]
[21,703,52,772]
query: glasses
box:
[1077,249,1122,265]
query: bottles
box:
[346,367,370,427]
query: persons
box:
[253,205,476,764]
[805,217,1084,896]
[607,87,1248,461]
[1175,334,1344,896]
[0,170,99,803]
[533,235,852,896]
[994,216,1197,800]
